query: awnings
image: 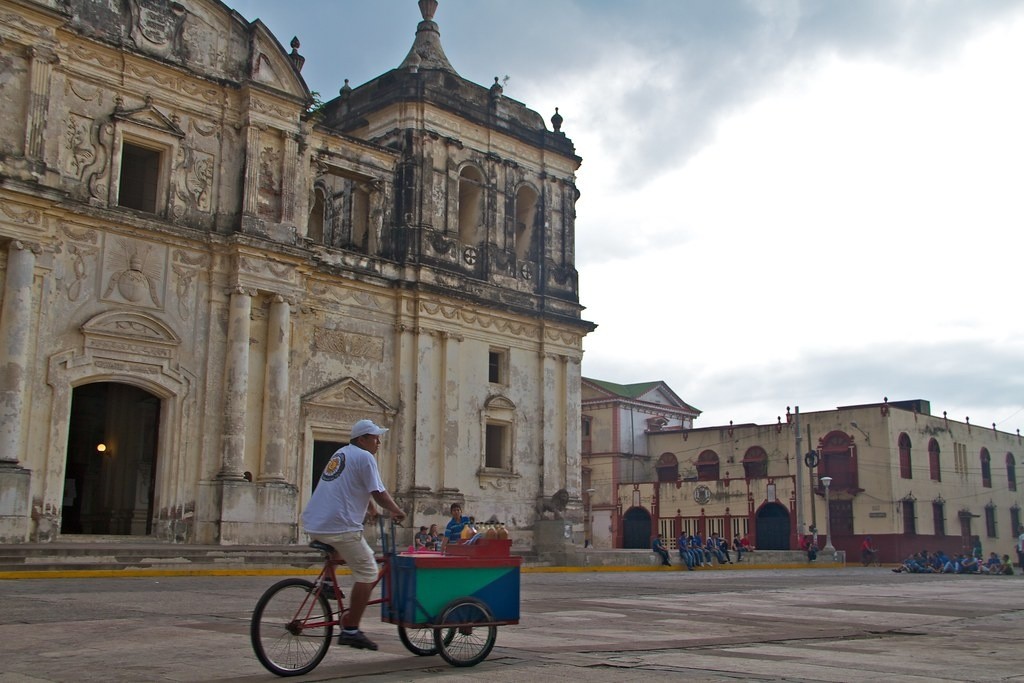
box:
[820,443,855,456]
[652,463,678,468]
[738,456,767,463]
[692,461,719,466]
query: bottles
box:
[461,520,508,540]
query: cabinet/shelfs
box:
[381,554,523,628]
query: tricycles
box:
[254,514,523,677]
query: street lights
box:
[821,477,835,551]
[587,489,596,548]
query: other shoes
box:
[687,562,712,571]
[892,568,901,572]
[662,561,672,566]
[720,560,727,564]
[729,561,732,563]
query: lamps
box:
[97,444,111,455]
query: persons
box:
[678,532,733,570]
[732,533,746,562]
[858,536,880,567]
[891,526,1024,577]
[801,535,818,563]
[652,534,673,567]
[440,503,477,558]
[740,533,756,552]
[302,419,408,651]
[415,523,445,553]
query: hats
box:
[351,419,388,439]
[420,526,428,530]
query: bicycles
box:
[861,546,882,567]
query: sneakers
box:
[338,631,377,650]
[318,583,345,600]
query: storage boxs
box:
[447,538,513,558]
